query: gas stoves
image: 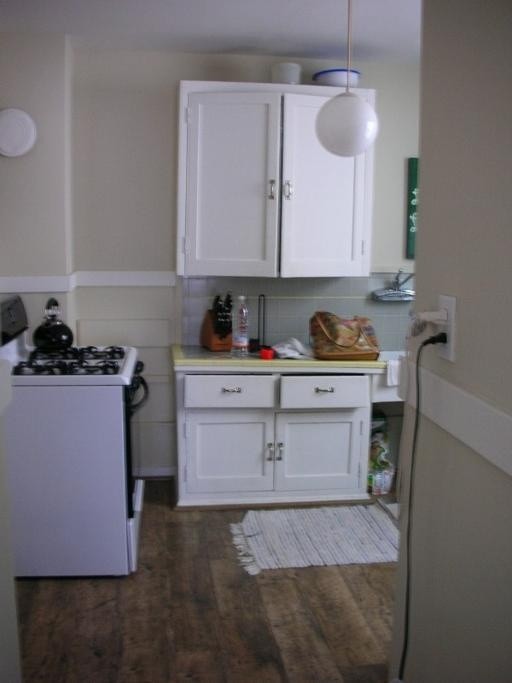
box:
[0,331,144,403]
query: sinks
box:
[372,351,406,403]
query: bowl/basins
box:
[313,68,361,86]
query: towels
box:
[384,360,400,387]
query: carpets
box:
[227,502,401,576]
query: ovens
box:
[0,376,149,575]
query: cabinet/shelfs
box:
[170,343,371,506]
[171,77,381,279]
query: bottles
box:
[233,296,250,356]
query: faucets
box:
[392,268,414,290]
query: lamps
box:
[313,2,380,159]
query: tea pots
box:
[33,298,73,350]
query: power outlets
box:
[432,292,458,366]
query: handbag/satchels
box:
[307,308,381,363]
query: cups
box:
[270,61,301,84]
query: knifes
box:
[212,293,234,337]
